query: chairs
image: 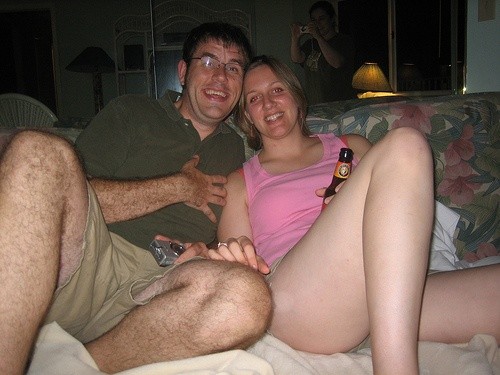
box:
[1,93,59,128]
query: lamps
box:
[350,62,392,93]
[66,46,115,114]
[397,62,430,91]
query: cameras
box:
[149,239,185,267]
[299,26,311,33]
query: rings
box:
[218,242,227,248]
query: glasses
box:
[190,55,246,74]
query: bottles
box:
[320,148,353,214]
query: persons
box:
[209,56,499,375]
[0,23,271,375]
[290,0,356,102]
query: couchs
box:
[0,91,500,375]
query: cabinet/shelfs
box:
[113,0,252,98]
